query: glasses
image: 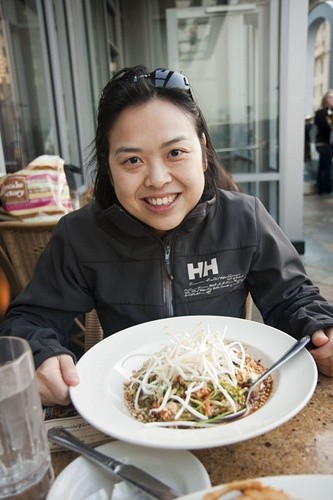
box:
[108,70,189,90]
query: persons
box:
[0,64,333,406]
[314,89,333,195]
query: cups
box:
[0,336,55,500]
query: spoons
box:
[212,335,310,420]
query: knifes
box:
[46,426,181,500]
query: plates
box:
[69,315,318,448]
[175,474,333,500]
[46,441,211,500]
